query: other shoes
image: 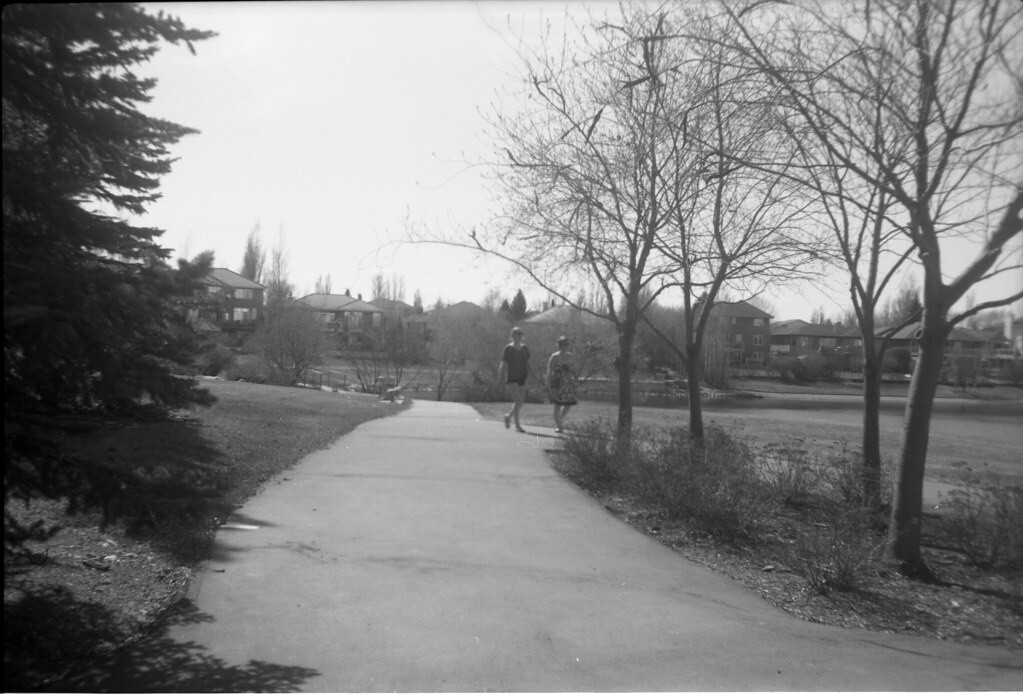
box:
[504,413,510,428]
[555,425,561,433]
[516,426,524,432]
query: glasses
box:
[515,331,524,335]
[561,343,569,347]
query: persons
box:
[499,327,535,432]
[546,336,578,433]
